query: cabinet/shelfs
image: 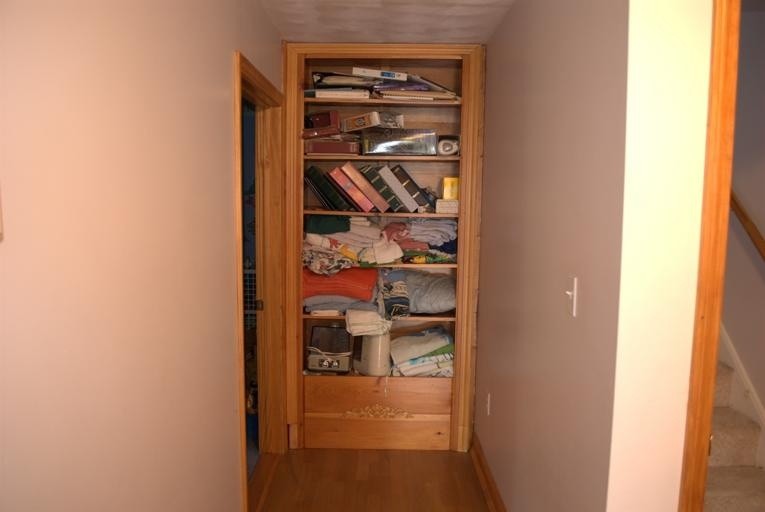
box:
[279,39,486,453]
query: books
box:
[304,66,461,103]
[304,162,436,213]
